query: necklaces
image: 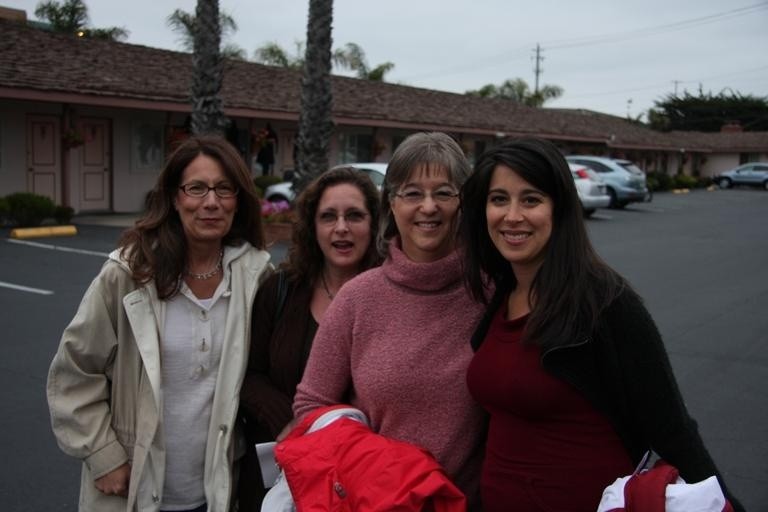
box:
[177,247,225,283]
[318,272,338,306]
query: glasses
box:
[394,191,459,202]
[317,211,369,224]
[180,182,237,198]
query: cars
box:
[567,164,610,215]
[263,163,388,214]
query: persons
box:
[46,129,277,511]
[448,135,749,510]
[234,161,391,511]
[285,132,495,511]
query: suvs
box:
[713,162,767,192]
[564,155,648,211]
